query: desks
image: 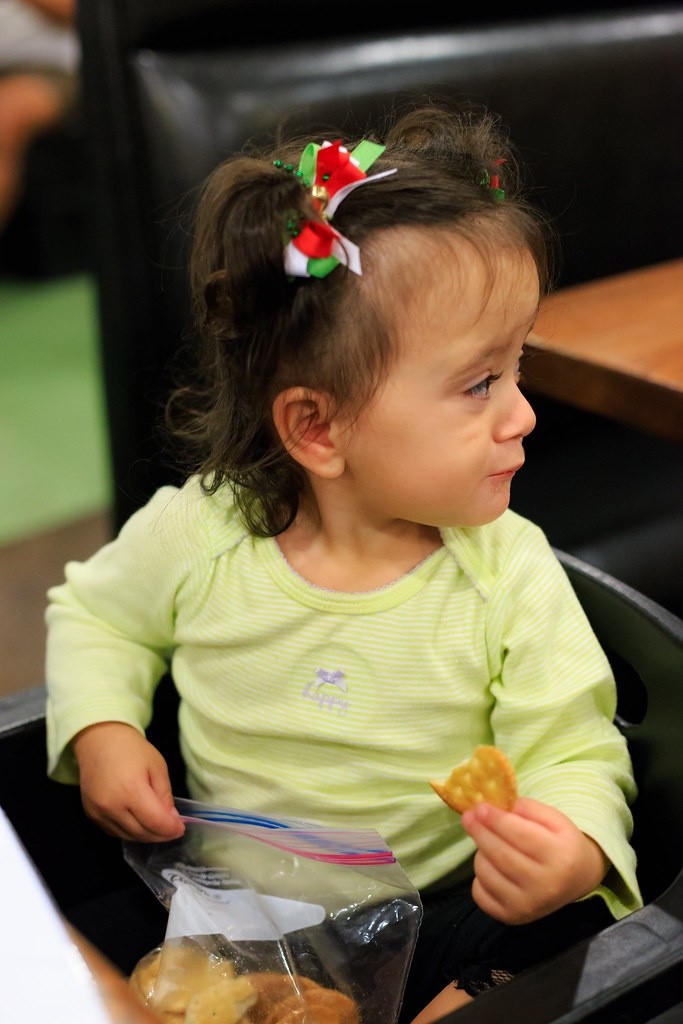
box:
[520,252,683,447]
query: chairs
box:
[130,10,680,797]
[0,547,683,1024]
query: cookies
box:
[427,745,517,820]
[127,946,362,1024]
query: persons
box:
[36,104,643,1024]
[0,1,172,470]
